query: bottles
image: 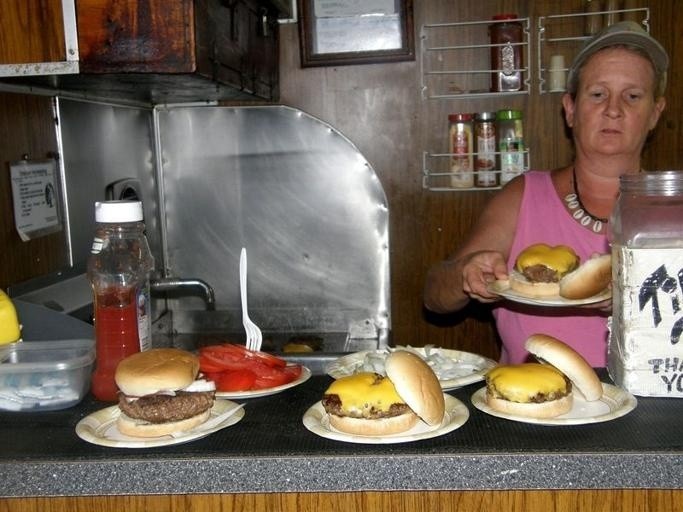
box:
[585,0,639,39]
[488,14,522,92]
[448,111,524,191]
[548,55,564,92]
[88,198,153,406]
[607,172,683,398]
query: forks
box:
[236,248,262,354]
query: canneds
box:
[448,109,526,188]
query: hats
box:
[570,20,668,74]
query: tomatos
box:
[195,344,300,391]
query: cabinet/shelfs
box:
[74,0,279,102]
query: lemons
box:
[0,289,21,346]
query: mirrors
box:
[296,0,415,68]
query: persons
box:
[422,19,670,369]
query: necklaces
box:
[567,161,643,231]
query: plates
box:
[325,345,498,393]
[467,382,636,426]
[72,394,247,449]
[302,391,469,447]
[485,274,612,315]
[217,362,309,400]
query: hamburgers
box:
[509,245,612,298]
[321,350,445,436]
[115,347,216,437]
[484,333,602,417]
